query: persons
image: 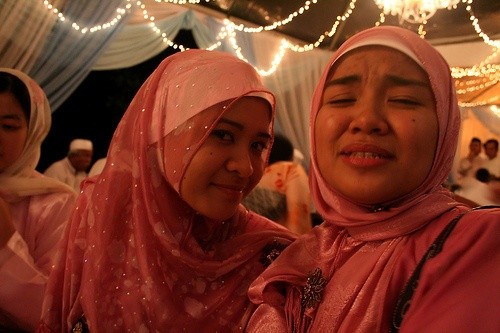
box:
[235,25,500,333]
[38,47,304,333]
[0,69,78,333]
[42,131,500,239]
